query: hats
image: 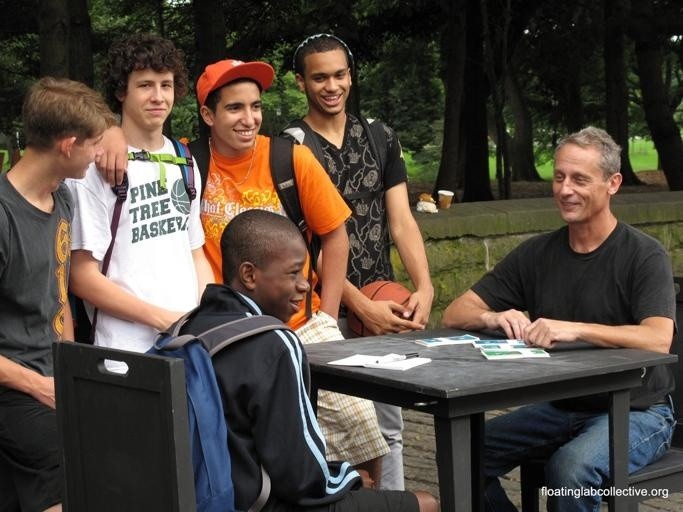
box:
[196,56,275,109]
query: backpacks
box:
[142,312,294,512]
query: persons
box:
[274,33,436,493]
[96,56,391,493]
[441,125,679,511]
[136,208,441,512]
[63,35,216,375]
[1,75,116,511]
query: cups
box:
[437,189,455,210]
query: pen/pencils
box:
[376,353,419,362]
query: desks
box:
[301,326,679,511]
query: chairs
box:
[519,276,683,511]
[50,338,199,512]
